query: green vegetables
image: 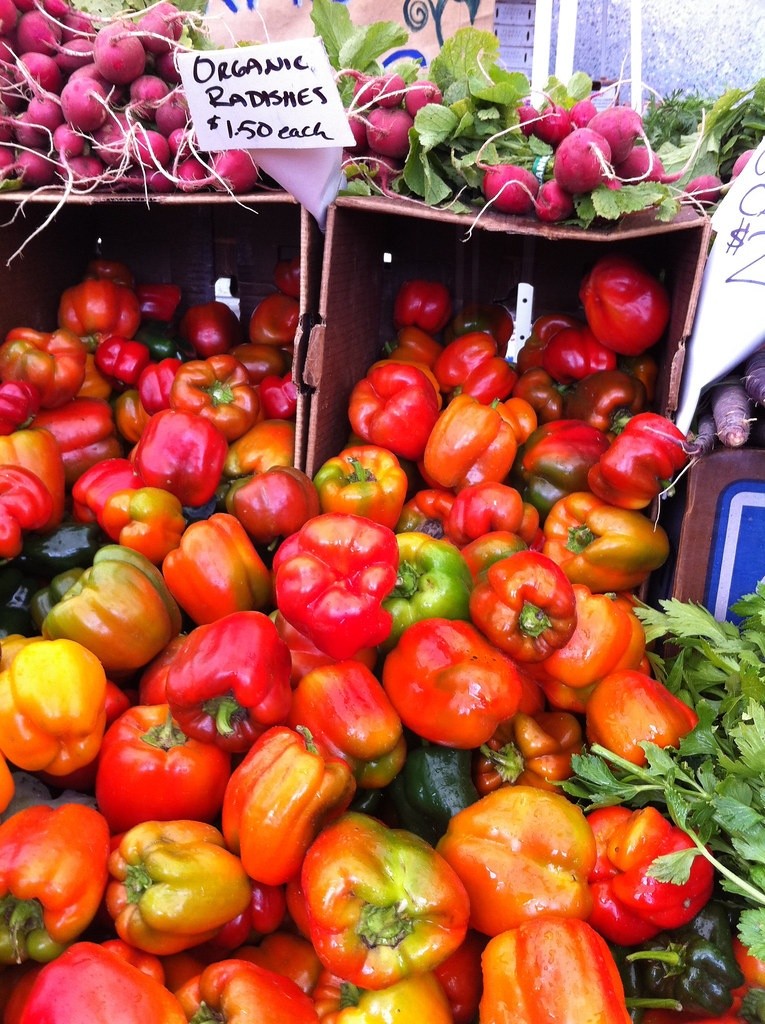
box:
[547,571,765,963]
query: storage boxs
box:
[0,188,712,604]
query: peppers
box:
[624,897,765,1024]
[0,254,689,1024]
